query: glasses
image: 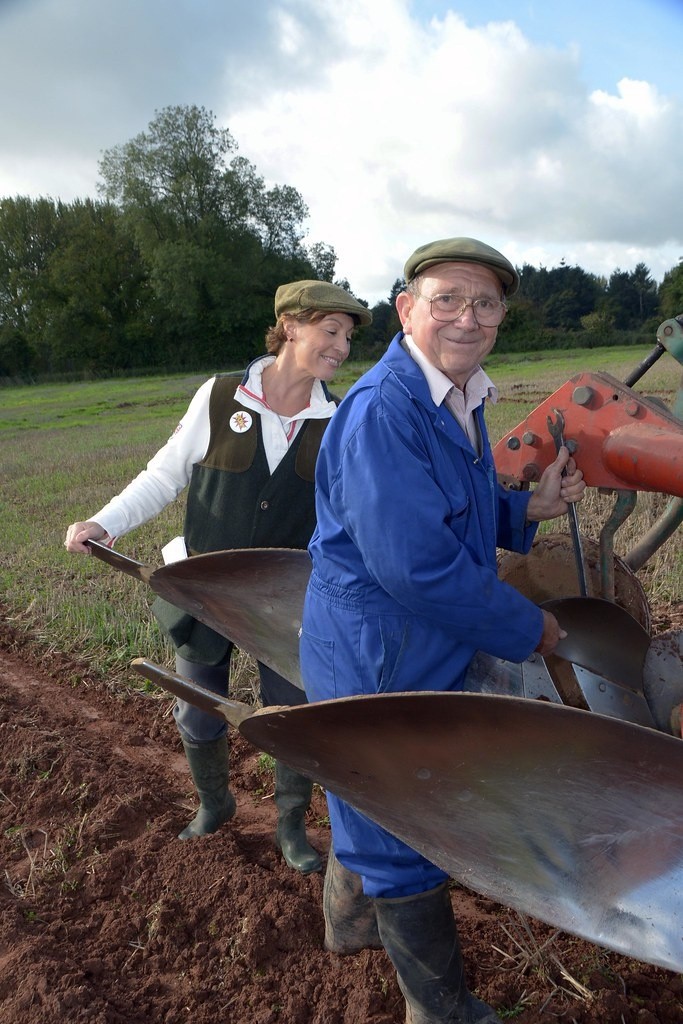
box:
[413,288,511,327]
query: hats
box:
[274,279,373,329]
[404,237,520,299]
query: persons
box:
[64,278,374,871]
[297,238,586,1024]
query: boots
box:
[323,838,502,1023]
[178,735,238,840]
[275,758,324,875]
